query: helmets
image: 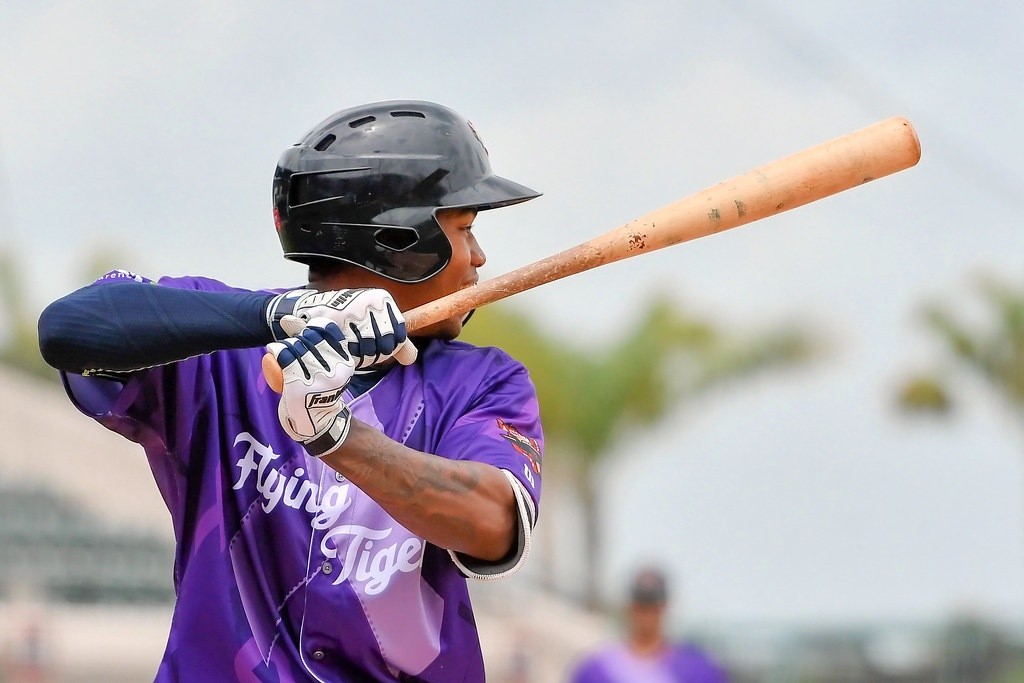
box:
[272,100,542,284]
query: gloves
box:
[265,315,355,457]
[266,288,418,371]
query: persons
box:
[564,561,734,683]
[35,96,548,683]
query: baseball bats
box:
[259,113,922,395]
